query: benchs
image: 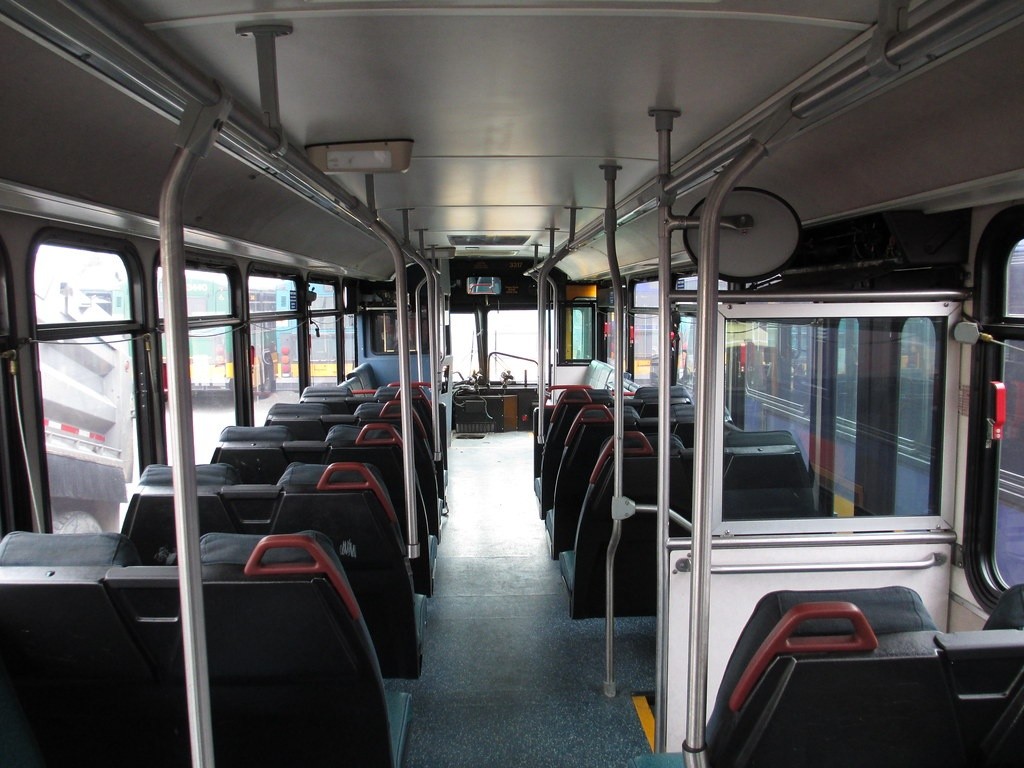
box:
[529,359,1024,768]
[0,362,452,767]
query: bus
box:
[78,282,276,401]
[572,246,1023,585]
[276,281,357,392]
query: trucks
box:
[34,254,134,534]
